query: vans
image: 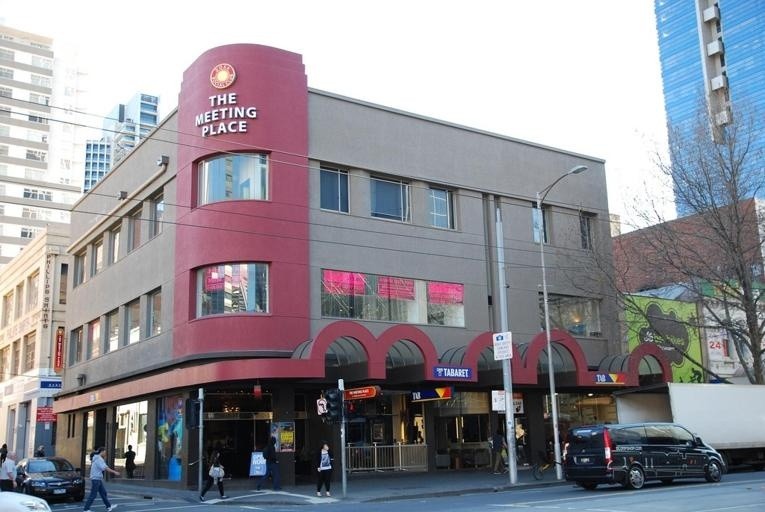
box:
[565,422,728,490]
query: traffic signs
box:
[343,385,381,401]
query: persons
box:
[0,443,18,492]
[312,439,335,497]
[199,440,229,503]
[89,444,104,461]
[34,445,45,457]
[122,445,136,478]
[491,423,531,475]
[82,447,118,512]
[254,436,282,492]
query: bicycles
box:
[533,441,569,481]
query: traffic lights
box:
[185,398,199,430]
[324,389,342,424]
[344,400,358,418]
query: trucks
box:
[611,381,765,470]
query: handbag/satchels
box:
[208,464,225,478]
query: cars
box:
[0,490,52,512]
[16,456,84,502]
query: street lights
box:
[534,165,589,482]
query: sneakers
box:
[326,492,331,497]
[84,510,93,512]
[107,504,118,512]
[317,492,322,497]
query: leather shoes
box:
[221,496,228,499]
